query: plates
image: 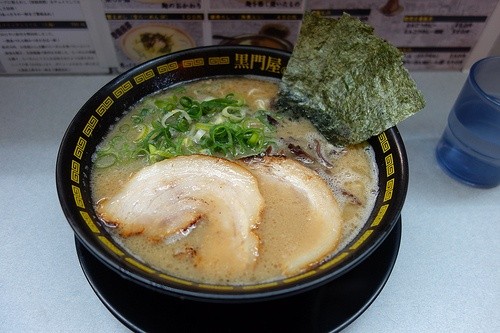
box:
[73,210,403,333]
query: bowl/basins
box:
[54,44,410,305]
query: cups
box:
[435,55,500,190]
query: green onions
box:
[91,85,282,168]
